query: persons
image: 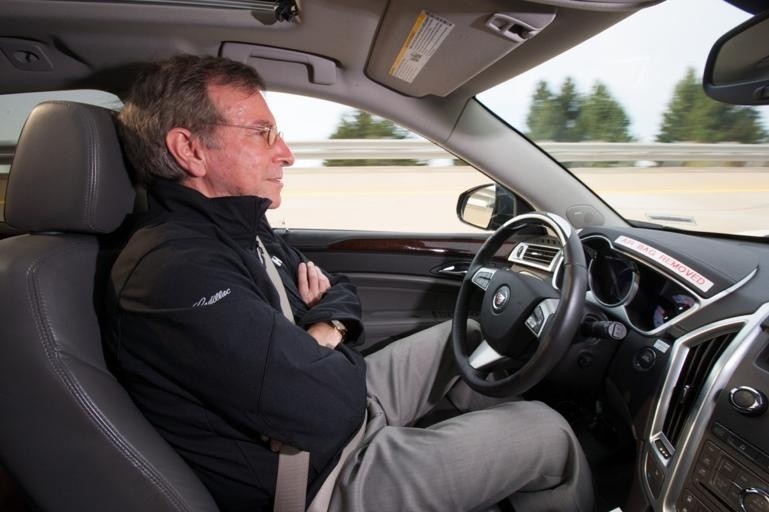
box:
[102,50,598,511]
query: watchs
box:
[329,319,350,338]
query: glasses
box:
[211,121,283,145]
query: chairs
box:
[0,100,221,512]
